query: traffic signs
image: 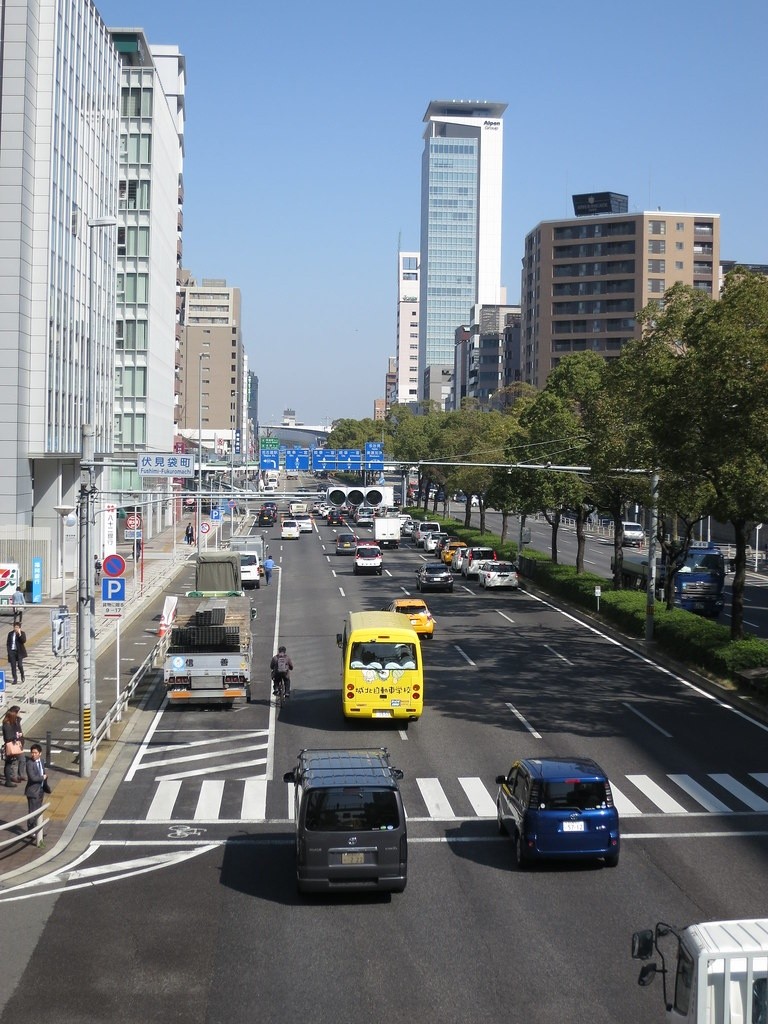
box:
[312,441,384,470]
[285,449,310,471]
[260,449,280,471]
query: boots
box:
[10,760,21,783]
[18,761,28,780]
[4,767,17,787]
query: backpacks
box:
[274,655,289,676]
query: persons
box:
[12,587,27,627]
[24,744,51,840]
[263,556,275,587]
[269,646,294,698]
[94,554,102,585]
[132,541,141,563]
[2,706,28,787]
[185,523,193,546]
[7,622,28,685]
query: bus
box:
[336,611,423,720]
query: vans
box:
[282,746,408,895]
[494,757,620,873]
[621,521,644,547]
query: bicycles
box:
[270,668,288,710]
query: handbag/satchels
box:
[6,740,24,754]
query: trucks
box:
[371,516,401,550]
[611,541,729,615]
[162,590,258,705]
[630,919,768,1024]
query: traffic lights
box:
[327,487,396,508]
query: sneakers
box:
[28,834,37,839]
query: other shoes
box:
[22,678,25,682]
[283,693,290,696]
[268,584,272,587]
[273,691,278,694]
[12,680,17,685]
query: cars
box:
[224,468,499,592]
[479,561,521,590]
[415,562,455,594]
[379,599,437,640]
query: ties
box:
[36,761,41,773]
